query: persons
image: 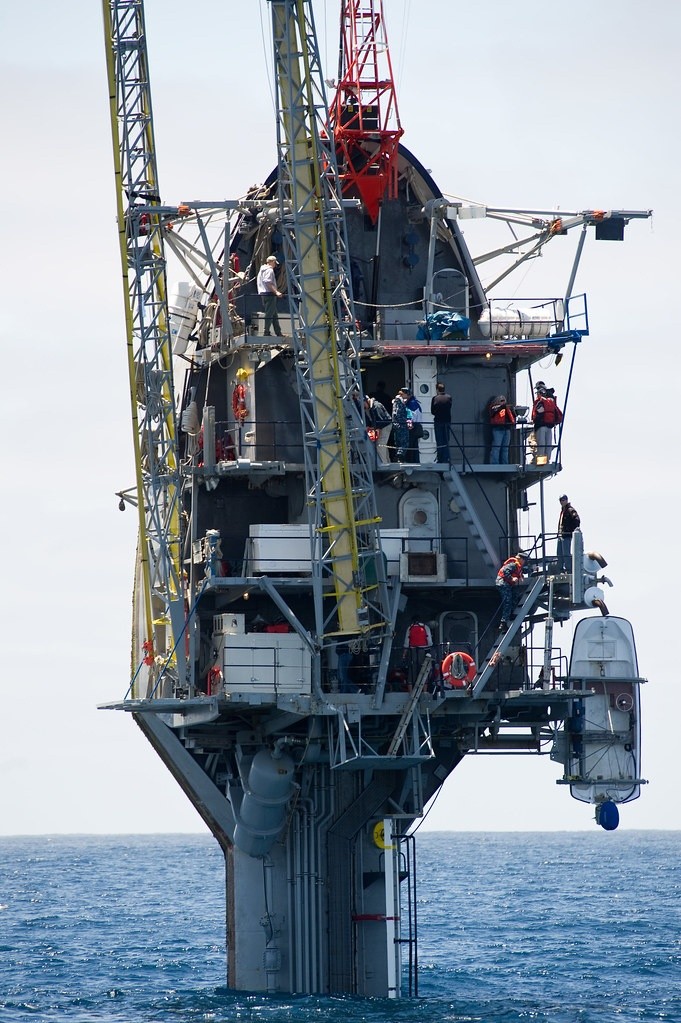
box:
[431,383,452,463]
[265,612,291,632]
[256,255,285,336]
[489,395,517,464]
[347,654,373,695]
[496,553,539,631]
[392,388,422,463]
[335,239,360,318]
[402,615,433,692]
[363,394,392,463]
[530,381,562,464]
[555,495,580,574]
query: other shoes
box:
[277,333,285,337]
[498,623,509,632]
[264,333,271,336]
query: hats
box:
[266,256,280,265]
[517,552,528,562]
[401,388,411,396]
[534,381,547,389]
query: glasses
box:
[500,400,506,402]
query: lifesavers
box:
[231,384,247,421]
[442,650,477,689]
